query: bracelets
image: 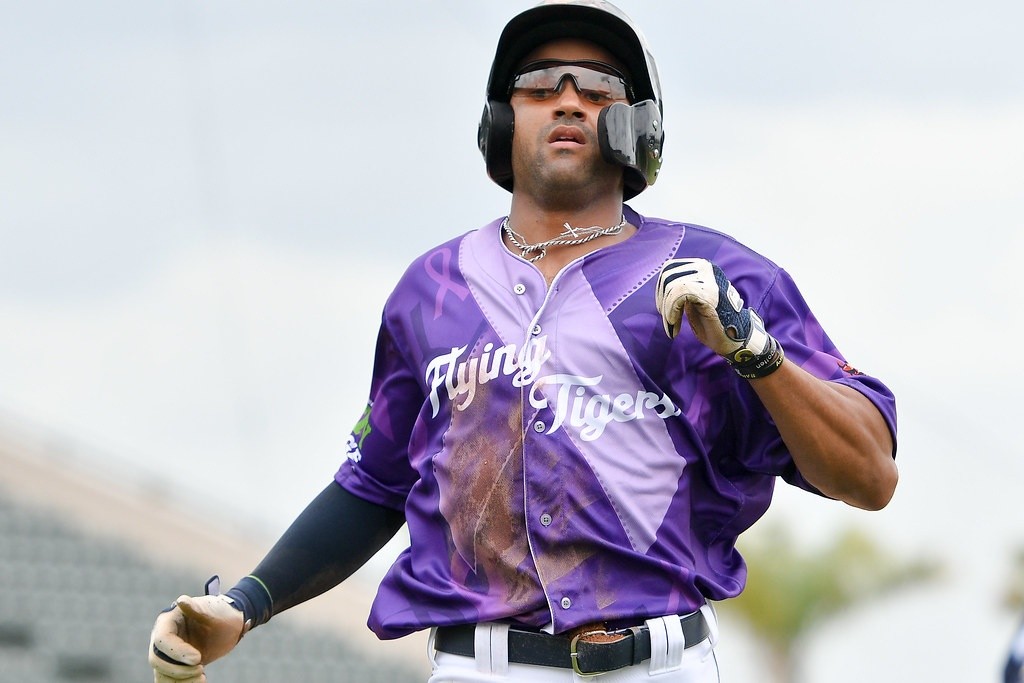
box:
[735,333,784,379]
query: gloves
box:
[147,575,252,683]
[655,256,785,380]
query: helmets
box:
[478,4,664,202]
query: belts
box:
[434,608,710,675]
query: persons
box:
[148,0,899,683]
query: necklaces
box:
[504,213,626,263]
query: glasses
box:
[506,58,636,105]
